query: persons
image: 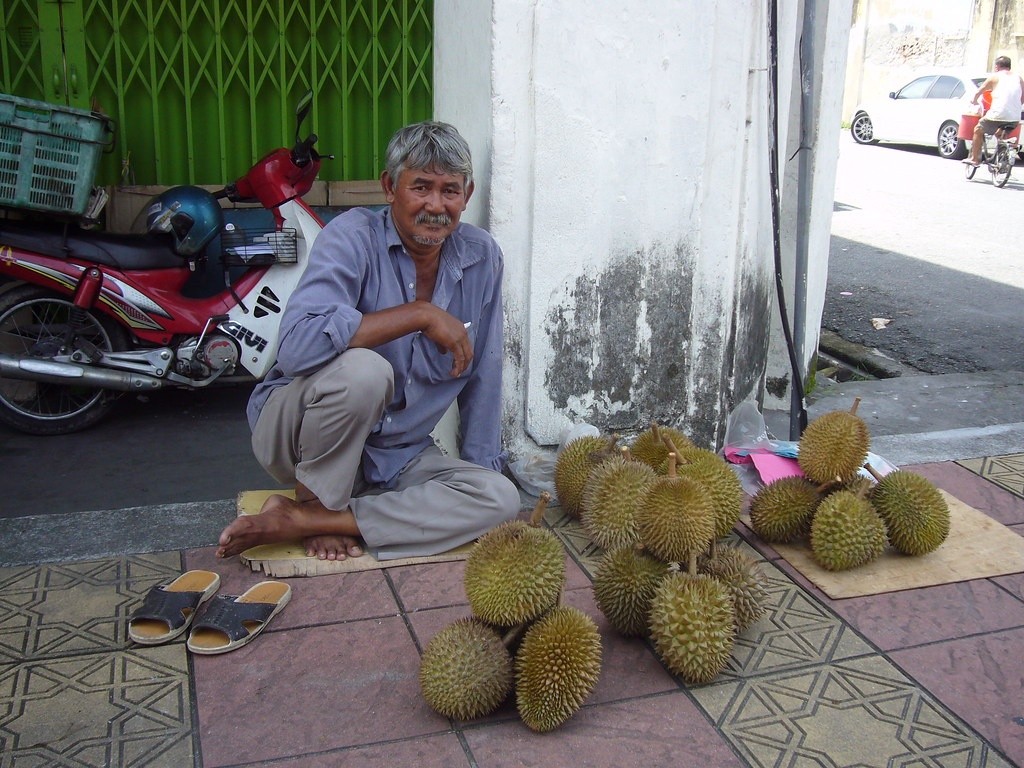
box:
[215,121,522,563]
[962,56,1024,165]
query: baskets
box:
[222,227,297,266]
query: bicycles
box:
[964,101,1020,187]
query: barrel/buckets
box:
[1004,123,1023,147]
[957,101,983,141]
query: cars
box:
[849,68,1024,159]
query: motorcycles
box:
[0,88,340,436]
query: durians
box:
[751,397,951,571]
[421,490,602,733]
[553,423,771,684]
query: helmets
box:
[147,185,225,259]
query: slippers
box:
[188,581,292,654]
[128,570,221,644]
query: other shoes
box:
[962,159,980,168]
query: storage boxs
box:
[1,92,115,215]
